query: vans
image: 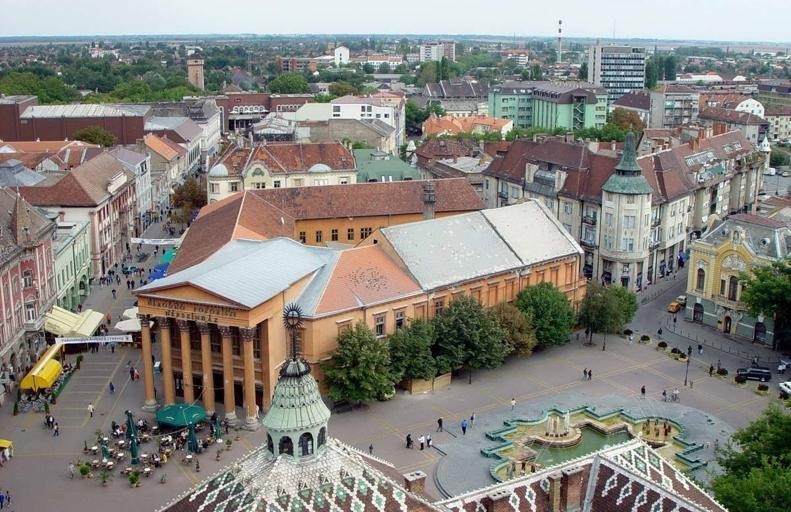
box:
[737,366,772,382]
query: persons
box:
[47,414,54,430]
[92,343,96,354]
[94,204,200,299]
[44,413,48,426]
[150,452,168,468]
[708,363,715,377]
[697,343,703,354]
[717,359,722,371]
[403,411,476,451]
[583,367,587,382]
[110,382,115,394]
[176,435,187,450]
[658,328,663,338]
[688,345,693,356]
[54,423,59,436]
[511,398,517,410]
[209,423,215,438]
[107,313,112,325]
[111,342,115,353]
[100,328,106,346]
[103,324,109,336]
[68,462,76,480]
[660,389,668,402]
[111,421,122,436]
[88,403,95,418]
[137,418,148,434]
[134,367,139,383]
[5,491,11,507]
[585,327,590,340]
[130,367,135,381]
[640,385,646,399]
[210,412,217,425]
[78,306,82,312]
[151,355,155,366]
[368,443,374,454]
[672,387,680,401]
[587,369,592,382]
[95,343,100,352]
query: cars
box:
[781,359,791,369]
[778,381,791,395]
[124,266,138,274]
[769,168,791,177]
[667,294,687,314]
